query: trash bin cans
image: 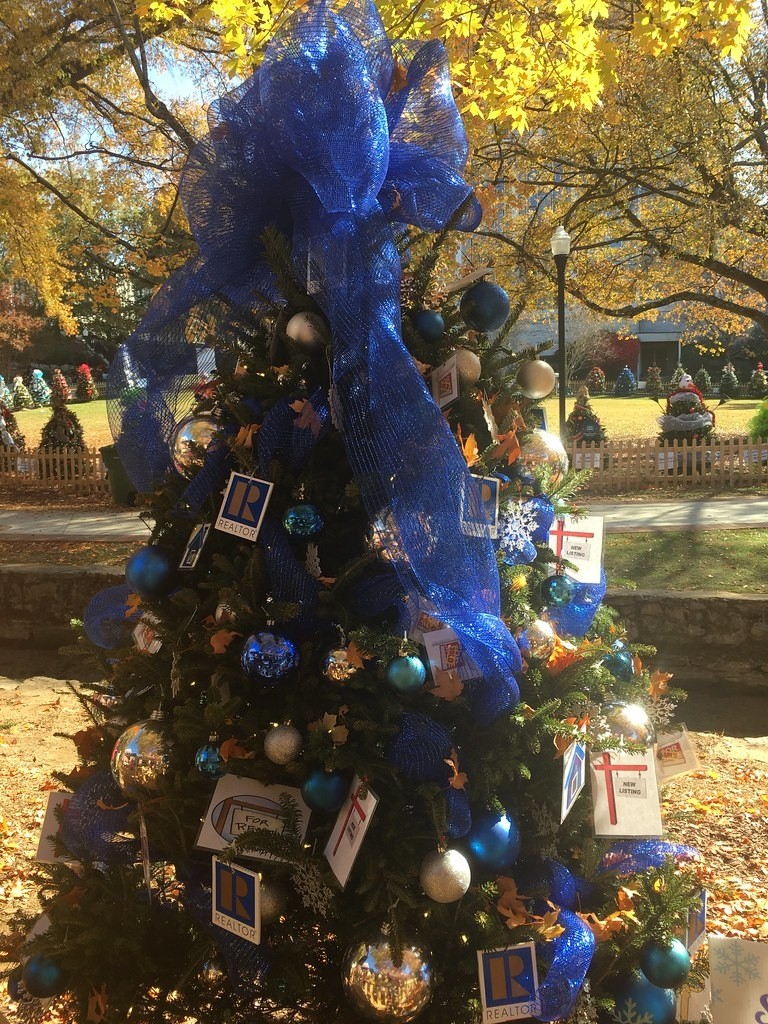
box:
[98,444,140,506]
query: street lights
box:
[549,224,570,451]
[677,331,683,366]
